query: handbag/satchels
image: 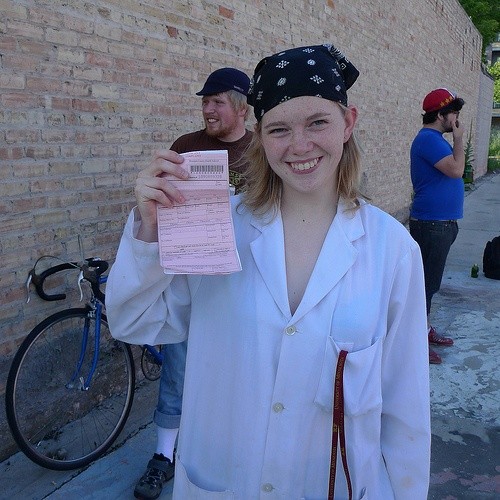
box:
[483,235,500,280]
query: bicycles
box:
[5,257,167,471]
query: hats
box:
[196,67,252,95]
[422,88,455,113]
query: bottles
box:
[471,264,479,278]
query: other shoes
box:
[427,325,454,346]
[134,448,177,500]
[429,345,441,364]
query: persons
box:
[409,87,466,364]
[105,42,432,499]
[134,67,255,500]
